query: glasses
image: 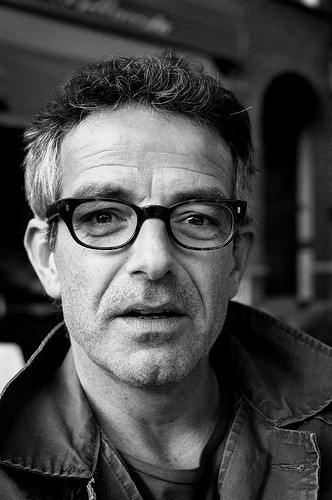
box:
[46,198,248,251]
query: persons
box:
[0,50,332,500]
